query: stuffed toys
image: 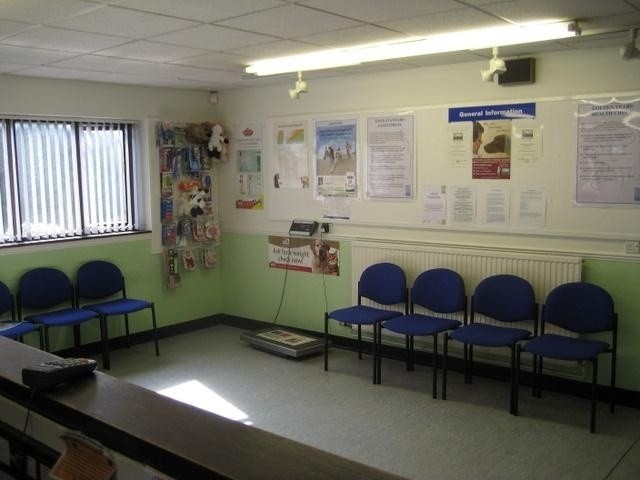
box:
[207,123,230,160]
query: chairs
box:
[440,274,540,414]
[376,268,468,400]
[0,260,160,372]
[322,263,408,384]
[512,282,617,435]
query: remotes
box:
[22,357,97,388]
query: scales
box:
[238,219,332,360]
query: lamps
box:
[287,72,308,101]
[620,29,639,62]
[479,48,507,85]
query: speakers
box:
[497,57,535,87]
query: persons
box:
[474,121,486,157]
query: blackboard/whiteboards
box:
[261,91,640,241]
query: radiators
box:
[350,240,586,371]
